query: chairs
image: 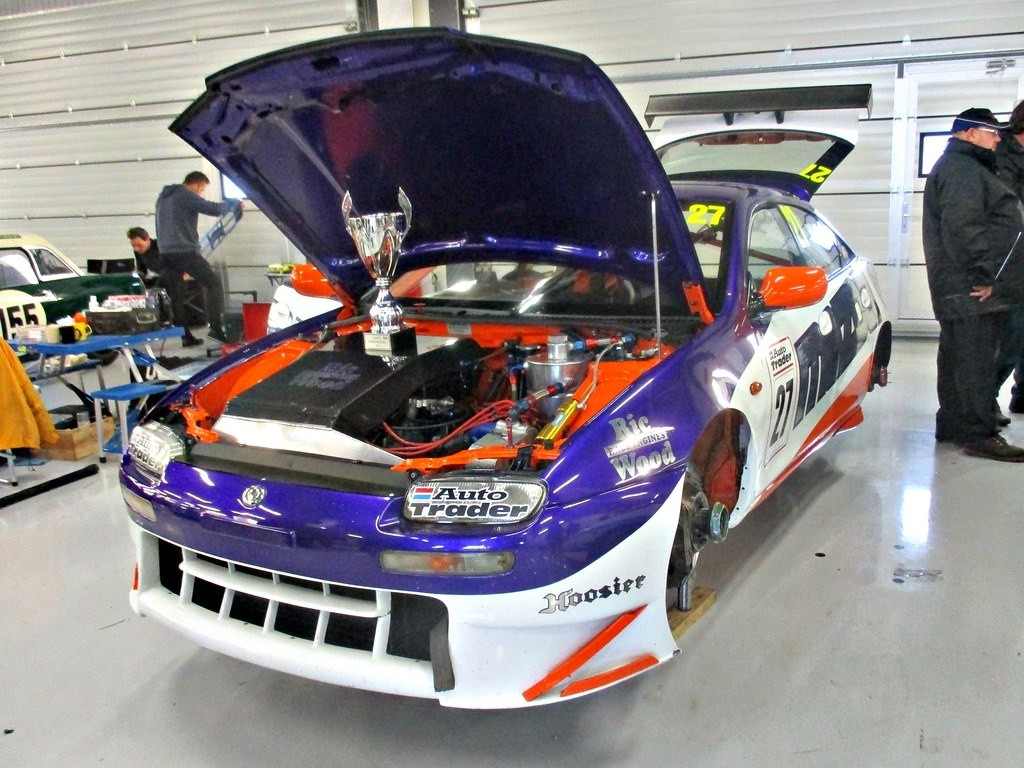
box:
[85,256,207,329]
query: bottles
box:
[88,295,99,311]
[72,312,86,323]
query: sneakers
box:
[180,333,203,347]
[207,327,226,344]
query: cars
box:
[0,231,119,367]
[117,26,894,711]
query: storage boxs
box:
[36,416,114,461]
[86,307,160,335]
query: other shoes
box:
[935,421,959,442]
[1009,397,1024,413]
[160,319,173,328]
[994,410,1011,426]
[963,434,1024,462]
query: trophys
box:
[341,186,414,352]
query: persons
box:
[126,228,190,289]
[923,101,1024,462]
[155,171,243,347]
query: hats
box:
[950,107,1011,135]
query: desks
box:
[6,324,186,456]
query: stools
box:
[92,383,164,465]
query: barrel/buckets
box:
[241,302,271,341]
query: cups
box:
[45,317,93,344]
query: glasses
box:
[963,126,1000,135]
